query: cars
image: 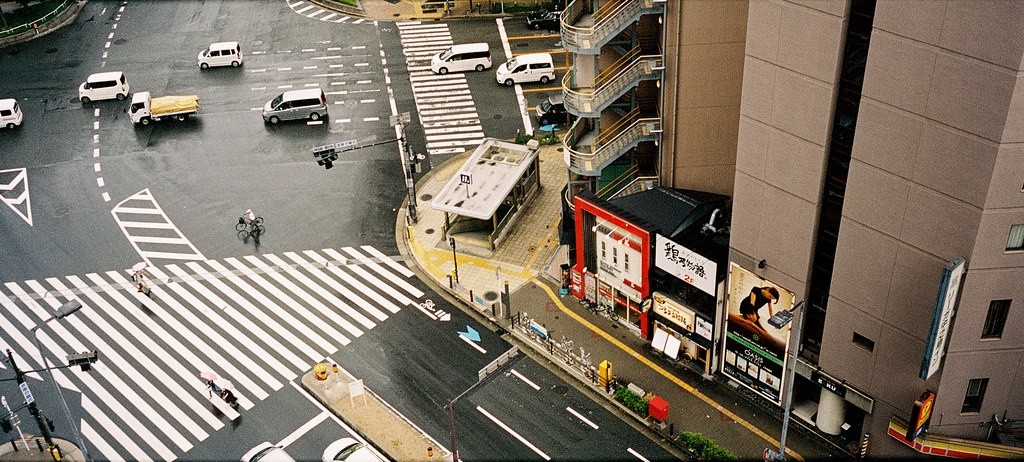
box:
[321,438,385,461]
[526,8,565,30]
[241,441,297,462]
[0,99,23,129]
[78,72,130,104]
[197,42,243,70]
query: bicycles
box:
[595,304,619,321]
[235,214,264,232]
[579,299,597,314]
[677,337,692,363]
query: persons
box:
[245,209,261,233]
[740,285,780,318]
[206,379,222,399]
[220,388,239,406]
[729,302,776,344]
[135,270,144,292]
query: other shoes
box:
[209,396,212,399]
[235,404,239,407]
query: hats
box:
[246,209,251,213]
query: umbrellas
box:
[132,261,146,272]
[200,371,217,381]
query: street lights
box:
[30,299,92,462]
[767,299,806,456]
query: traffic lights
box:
[449,237,454,248]
[317,153,338,170]
[410,163,415,173]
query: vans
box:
[431,43,492,75]
[534,94,573,126]
[496,53,556,87]
[262,88,328,124]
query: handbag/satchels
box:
[131,274,137,282]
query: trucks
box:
[127,92,201,126]
